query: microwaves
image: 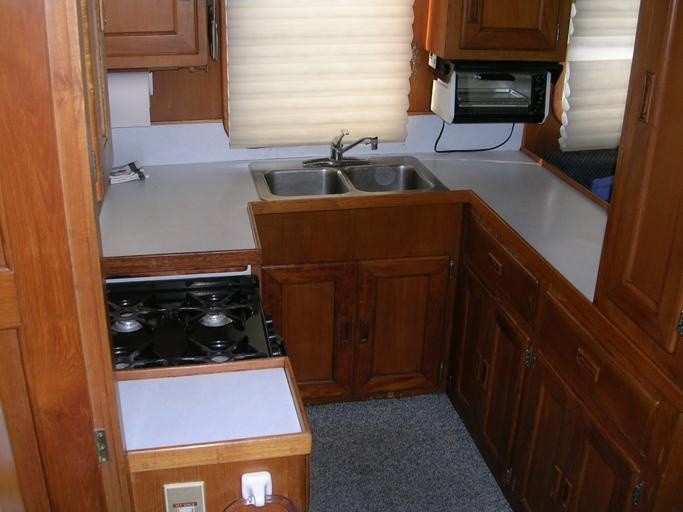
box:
[428,61,551,124]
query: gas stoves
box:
[104,268,283,372]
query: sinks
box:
[248,156,353,201]
[338,155,450,197]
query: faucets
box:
[329,129,378,161]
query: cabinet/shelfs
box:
[89,1,213,74]
[587,0,682,394]
[444,187,549,508]
[624,388,683,512]
[495,263,682,512]
[411,0,576,65]
[68,0,115,217]
[254,252,456,411]
